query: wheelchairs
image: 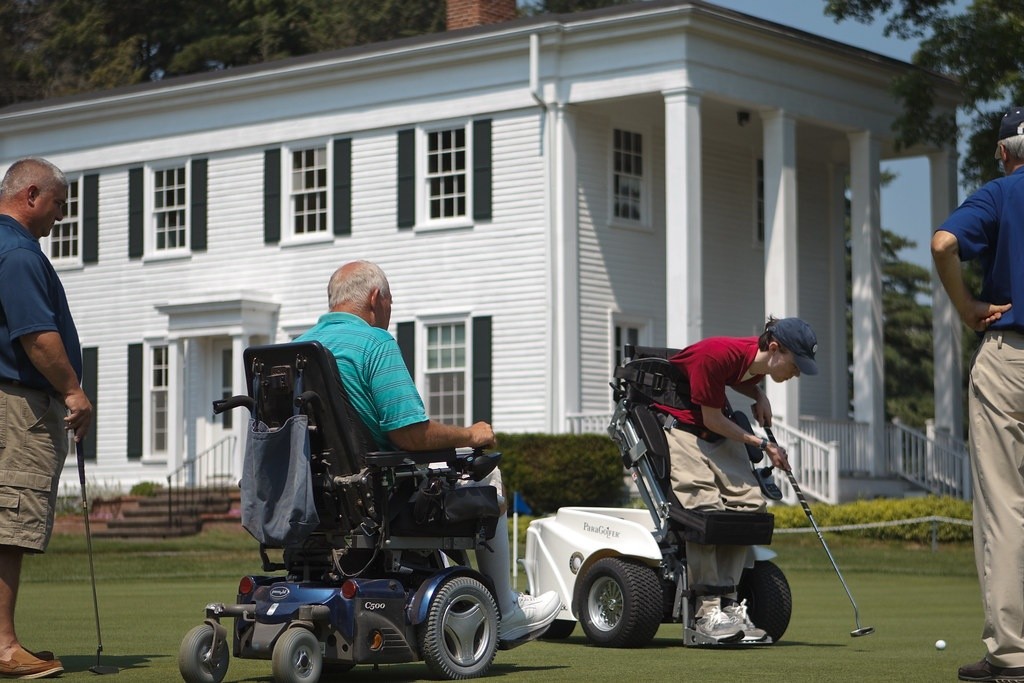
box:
[177,340,523,683]
[512,343,793,651]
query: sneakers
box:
[694,606,745,644]
[958,656,1024,683]
[722,598,767,642]
[499,590,562,640]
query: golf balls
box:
[934,640,946,650]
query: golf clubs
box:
[751,402,875,637]
[71,408,119,675]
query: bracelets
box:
[757,435,768,452]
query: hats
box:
[767,317,819,376]
[994,106,1024,159]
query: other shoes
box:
[0,645,64,679]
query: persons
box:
[663,315,821,650]
[1,156,95,683]
[291,259,565,643]
[929,103,1023,683]
